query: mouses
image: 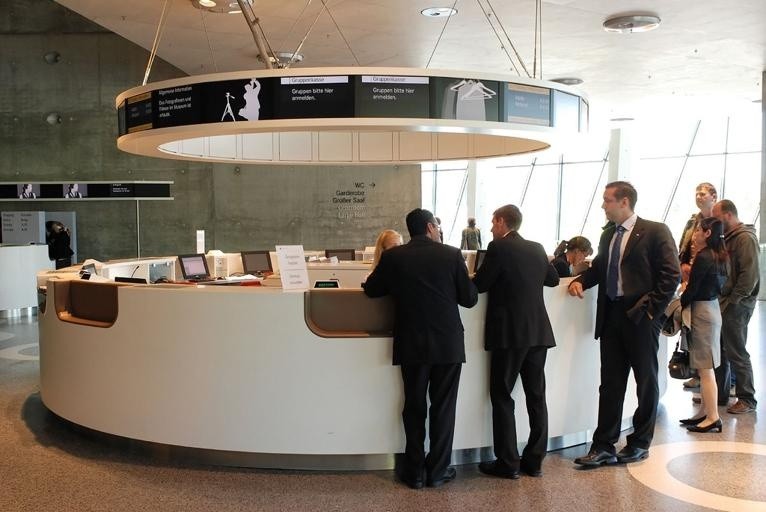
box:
[217,275,226,280]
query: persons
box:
[64,183,83,199]
[19,184,36,200]
[680,217,729,433]
[692,199,761,414]
[550,236,593,277]
[468,204,561,479]
[567,181,682,467]
[364,229,404,284]
[678,182,718,292]
[238,78,261,122]
[363,208,479,489]
[48,222,74,270]
[460,217,482,250]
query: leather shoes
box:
[425,467,456,486]
[692,393,726,405]
[574,445,617,466]
[407,480,422,489]
[727,400,755,414]
[616,443,649,463]
[478,460,519,479]
[520,459,543,477]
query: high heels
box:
[679,414,707,425]
[686,416,722,433]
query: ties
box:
[607,225,625,302]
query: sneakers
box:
[683,377,700,388]
[729,385,736,397]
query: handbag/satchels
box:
[668,329,691,379]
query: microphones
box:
[131,265,140,279]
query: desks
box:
[35,249,668,473]
[0,241,57,319]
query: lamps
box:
[191,0,255,15]
[601,16,661,33]
[553,79,584,86]
[256,52,303,66]
[421,8,459,17]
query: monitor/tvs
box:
[241,250,274,275]
[178,253,210,279]
[473,249,487,273]
[324,249,355,261]
